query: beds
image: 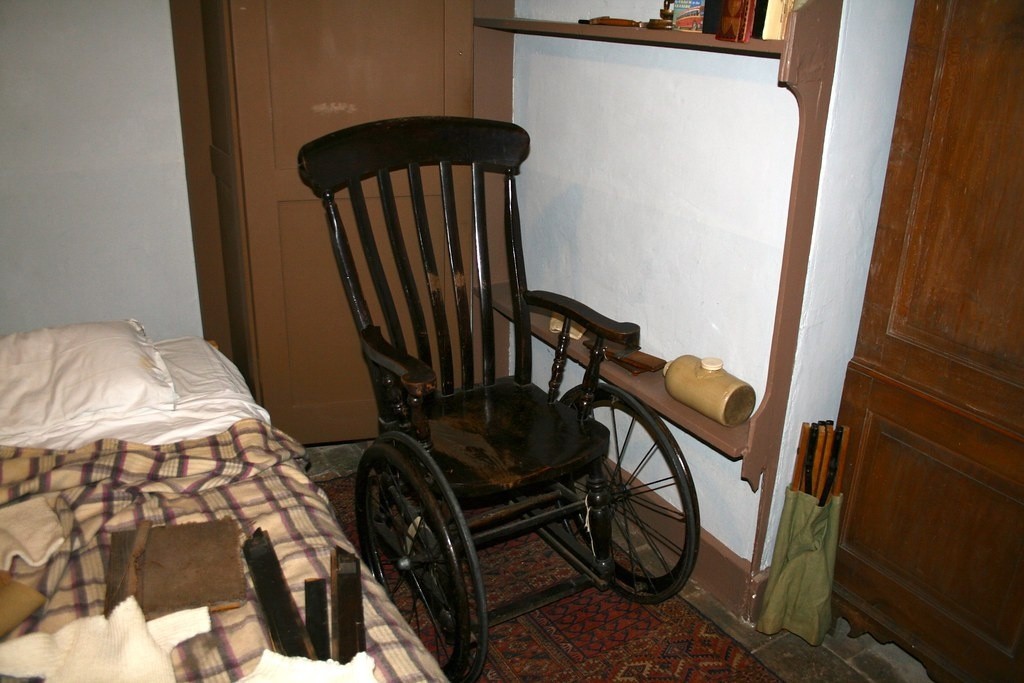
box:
[0,317,456,683]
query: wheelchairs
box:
[298,113,701,683]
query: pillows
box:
[0,317,177,435]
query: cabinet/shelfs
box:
[472,1,839,621]
